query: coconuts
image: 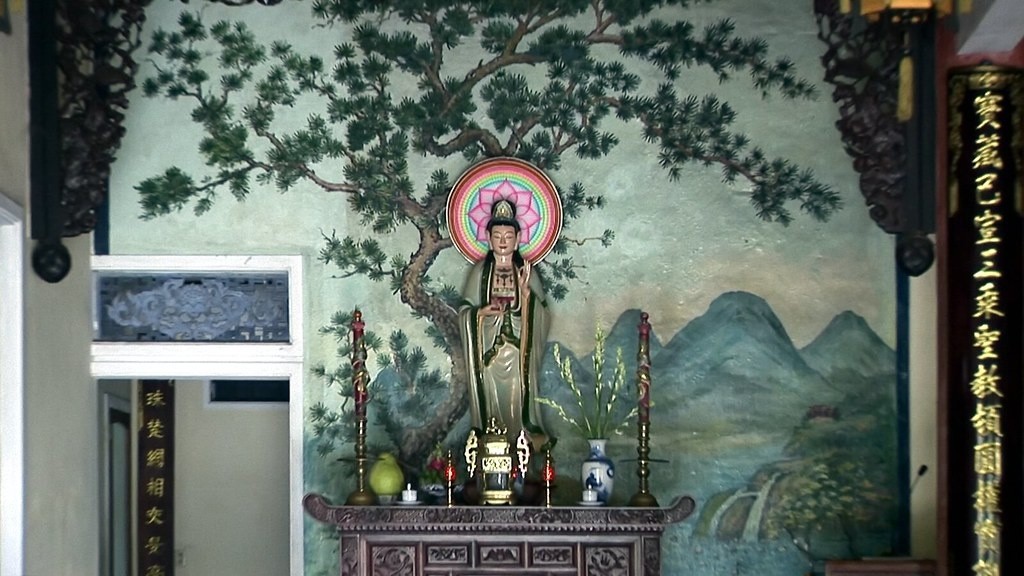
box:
[369,450,405,495]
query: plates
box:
[395,500,424,505]
[577,501,604,507]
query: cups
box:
[582,489,599,501]
[401,490,417,501]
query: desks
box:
[301,489,696,576]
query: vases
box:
[581,435,616,504]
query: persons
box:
[457,199,559,454]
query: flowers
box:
[531,312,655,439]
